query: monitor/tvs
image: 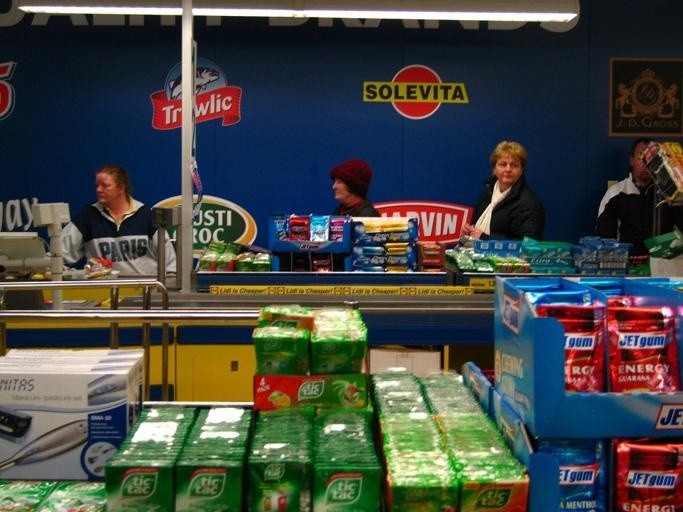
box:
[0,232,48,278]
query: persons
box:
[328,157,383,216]
[59,164,175,275]
[592,136,683,258]
[460,140,545,242]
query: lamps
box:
[12,0,582,23]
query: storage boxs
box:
[369,348,441,374]
[0,348,145,483]
[253,371,368,410]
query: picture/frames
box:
[609,57,683,137]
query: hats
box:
[328,158,372,200]
